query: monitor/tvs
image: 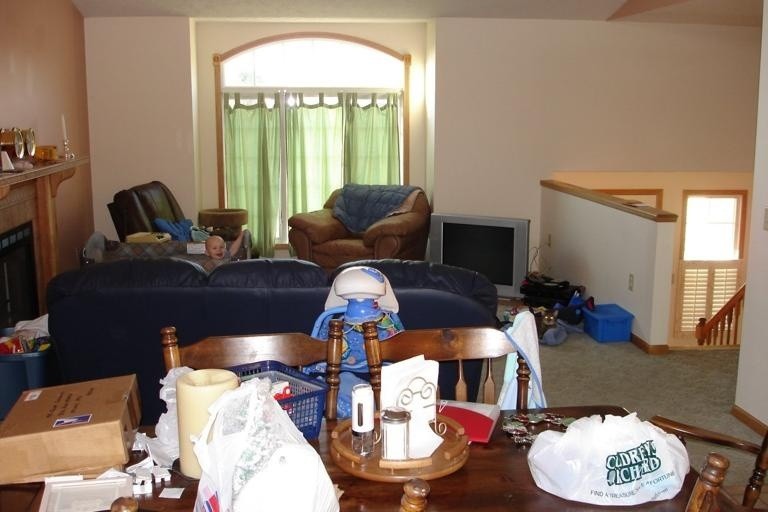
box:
[430,212,530,300]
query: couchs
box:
[45,256,498,428]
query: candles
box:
[60,112,67,140]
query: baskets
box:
[224,359,332,442]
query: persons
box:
[198,227,246,274]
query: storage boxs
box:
[581,303,636,345]
[0,372,145,486]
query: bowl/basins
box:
[36,146,58,161]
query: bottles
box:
[350,383,412,462]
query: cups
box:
[174,367,239,482]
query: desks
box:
[0,404,750,512]
